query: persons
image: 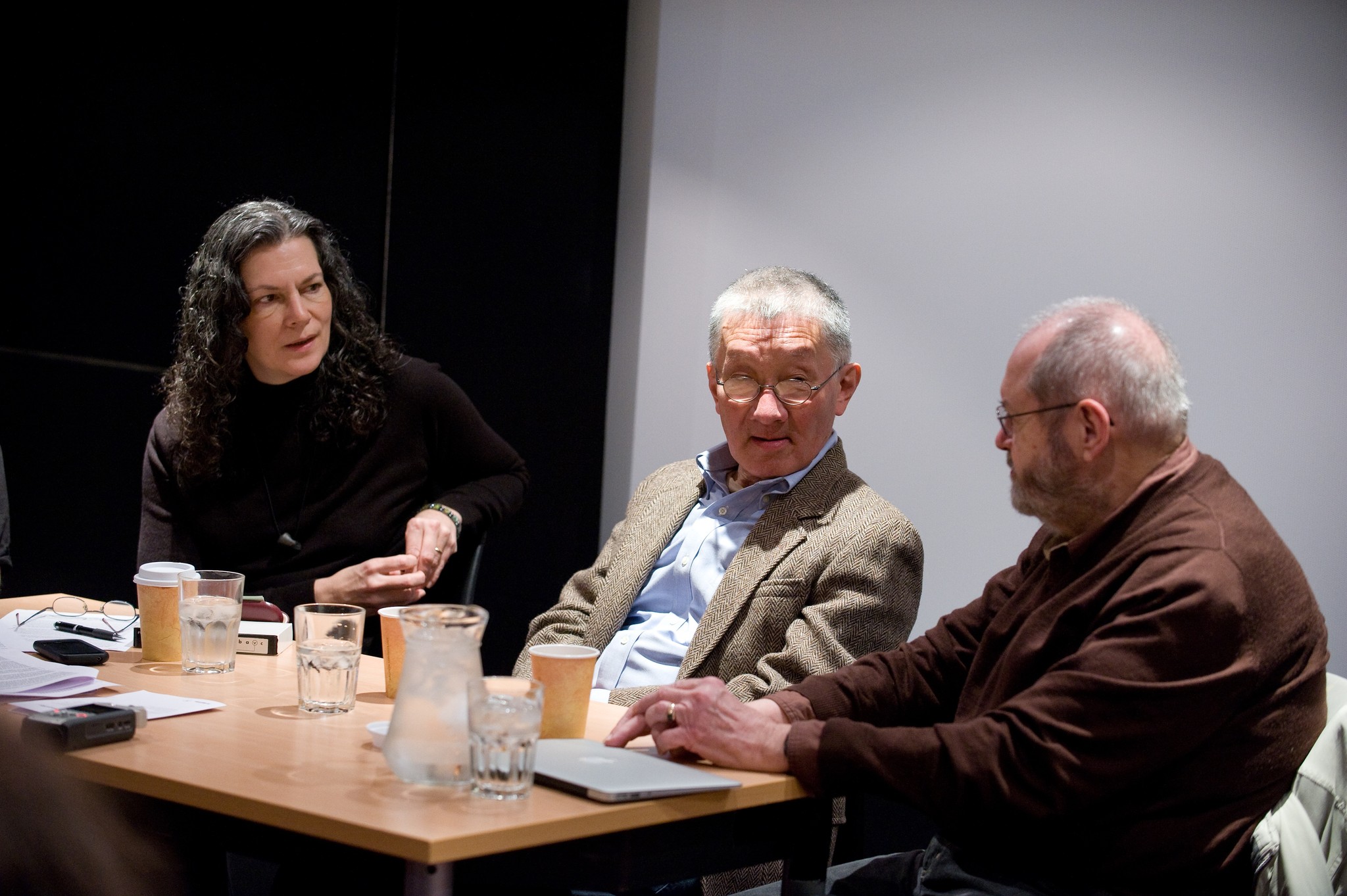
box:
[604,297,1328,896]
[512,266,924,896]
[137,197,532,659]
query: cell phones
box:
[33,638,110,666]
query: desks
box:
[0,594,831,896]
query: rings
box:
[667,703,677,722]
[435,547,442,555]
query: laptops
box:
[491,736,744,804]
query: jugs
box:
[383,604,489,786]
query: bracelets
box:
[421,503,461,538]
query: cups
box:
[529,644,600,739]
[377,607,411,699]
[133,562,201,662]
[293,603,366,715]
[316,659,318,660]
[467,675,545,801]
[177,570,245,674]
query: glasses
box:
[995,405,1115,438]
[715,363,846,405]
[16,596,139,633]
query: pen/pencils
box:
[53,620,126,641]
